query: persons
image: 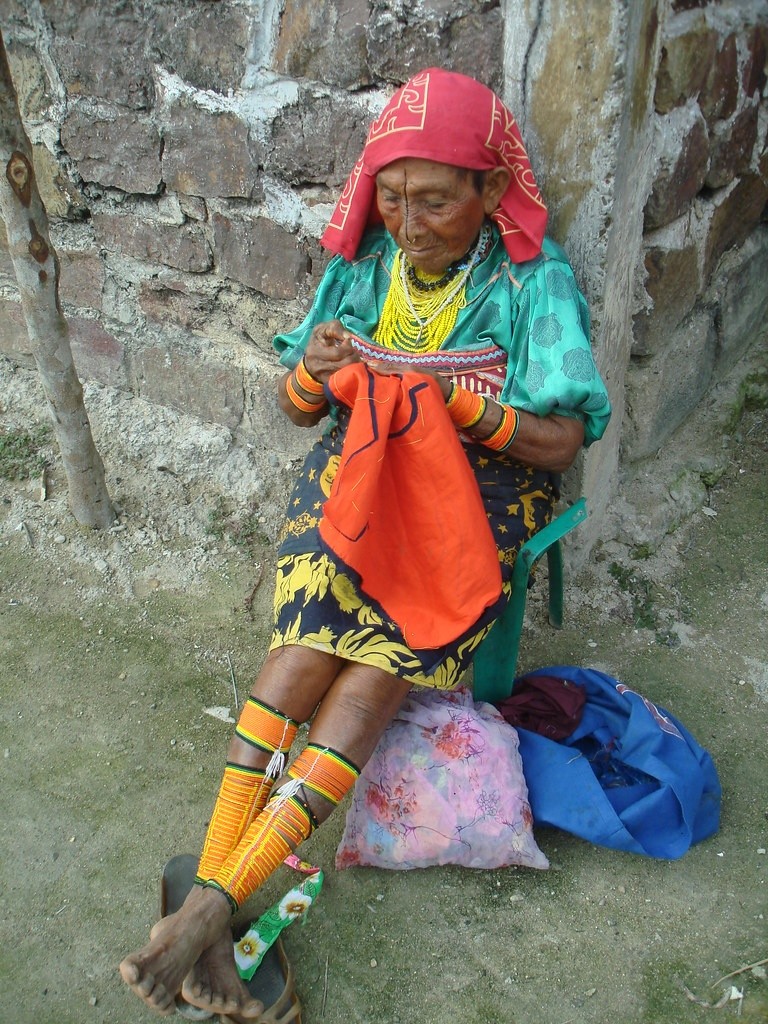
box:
[120,67,611,1020]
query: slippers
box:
[217,917,302,1024]
[159,854,214,1021]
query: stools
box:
[471,495,592,705]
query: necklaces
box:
[374,224,492,355]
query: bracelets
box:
[473,397,519,453]
[445,380,488,431]
[294,354,325,396]
[284,375,328,413]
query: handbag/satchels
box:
[497,664,720,861]
[335,685,551,871]
